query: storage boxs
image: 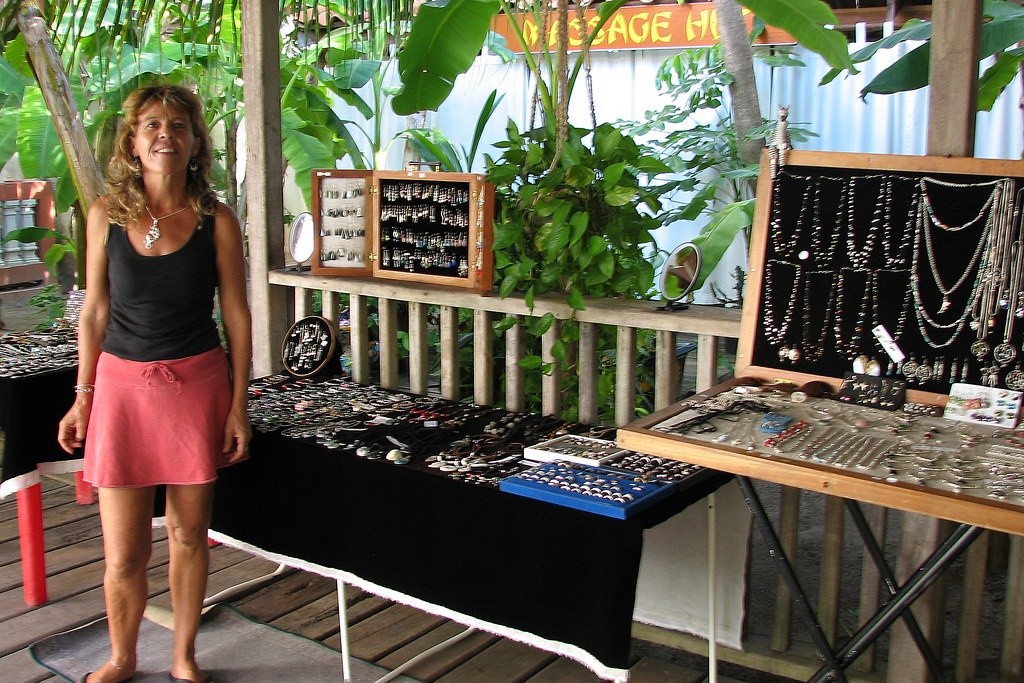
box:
[499,431,719,522]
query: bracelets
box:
[75,384,95,393]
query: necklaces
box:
[764,171,1024,364]
[143,204,191,250]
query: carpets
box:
[26,604,417,683]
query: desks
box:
[0,327,96,605]
[615,381,1024,683]
[142,377,734,683]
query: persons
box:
[59,84,251,683]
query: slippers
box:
[80,672,134,683]
[168,672,212,683]
